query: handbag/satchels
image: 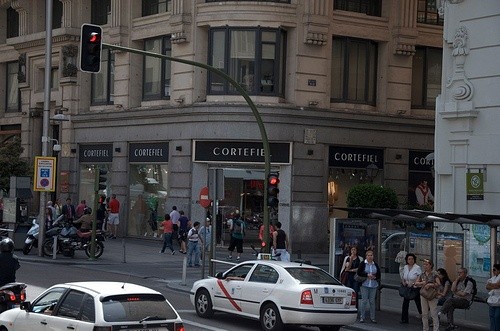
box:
[419,285,439,299]
[487,291,499,304]
[354,260,367,282]
[340,269,349,284]
[398,284,419,300]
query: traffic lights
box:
[79,23,102,74]
[266,171,280,207]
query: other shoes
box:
[415,314,422,318]
[399,320,409,324]
[371,320,377,323]
[359,317,364,322]
[445,323,454,331]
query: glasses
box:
[493,269,497,271]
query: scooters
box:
[0,282,30,311]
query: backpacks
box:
[456,276,477,296]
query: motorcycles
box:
[23,214,110,258]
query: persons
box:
[160,205,190,256]
[0,238,20,289]
[260,220,289,261]
[132,195,154,237]
[96,194,120,241]
[187,217,212,268]
[440,267,473,331]
[400,253,422,325]
[436,268,452,306]
[416,178,434,206]
[416,258,441,331]
[227,213,246,262]
[340,245,364,310]
[485,263,500,331]
[46,198,93,232]
[357,248,381,324]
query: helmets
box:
[0,238,14,252]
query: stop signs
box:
[199,186,211,207]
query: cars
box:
[0,281,185,331]
[189,259,358,331]
